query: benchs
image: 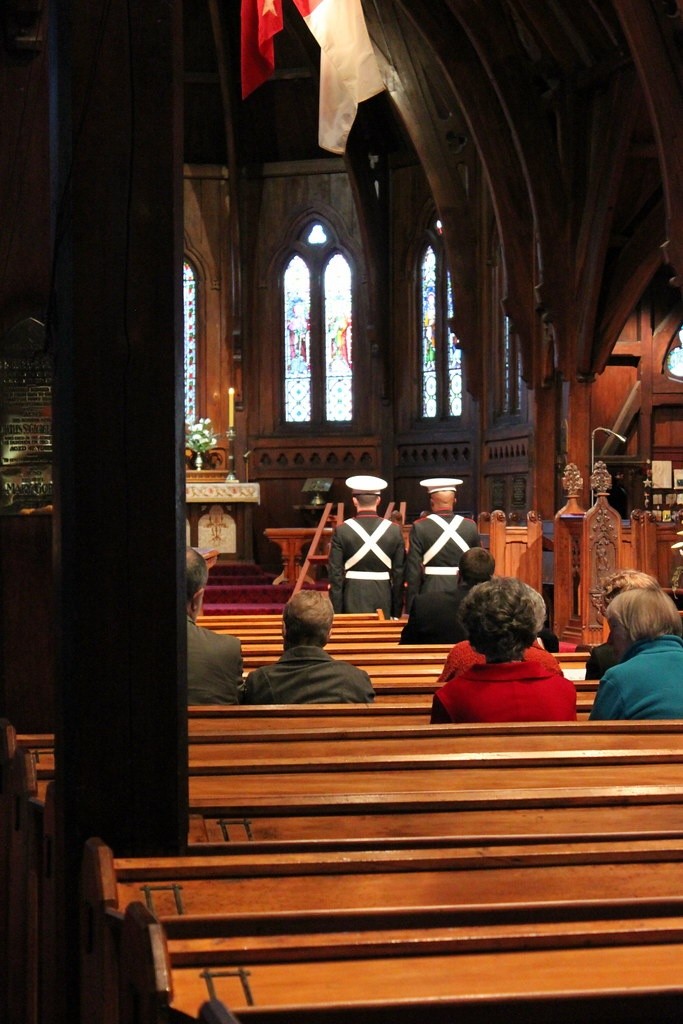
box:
[0,609,683,1024]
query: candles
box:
[228,387,235,427]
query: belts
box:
[423,567,459,575]
[346,570,391,580]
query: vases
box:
[195,452,203,471]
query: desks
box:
[264,528,333,585]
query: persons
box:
[245,590,375,705]
[329,475,406,621]
[186,547,245,705]
[398,547,683,724]
[403,478,481,617]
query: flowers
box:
[185,414,218,452]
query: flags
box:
[240,1,386,154]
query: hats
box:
[420,478,463,493]
[345,475,387,495]
